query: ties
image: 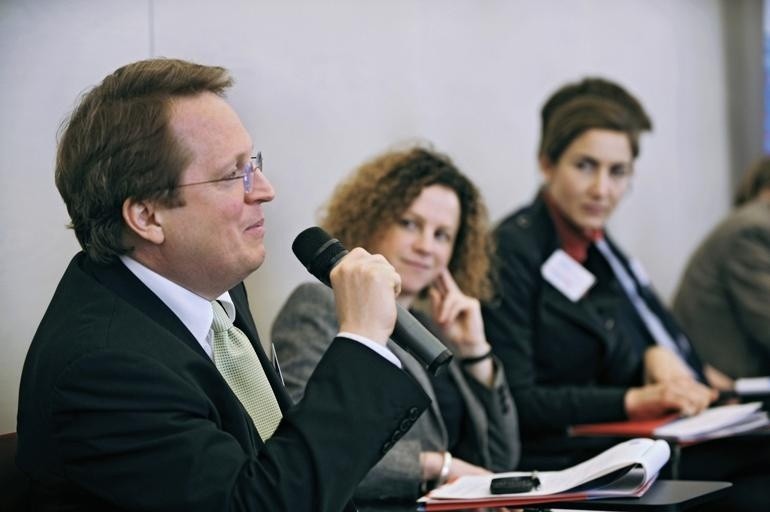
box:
[206,298,284,444]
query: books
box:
[566,399,769,445]
[415,437,672,512]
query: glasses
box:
[140,150,264,193]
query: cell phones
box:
[491,476,536,493]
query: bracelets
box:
[419,446,433,490]
[460,340,495,368]
[437,446,453,486]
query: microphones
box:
[291,226,454,376]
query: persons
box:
[537,74,739,418]
[670,153,769,385]
[270,145,527,512]
[12,58,437,511]
[475,96,716,477]
